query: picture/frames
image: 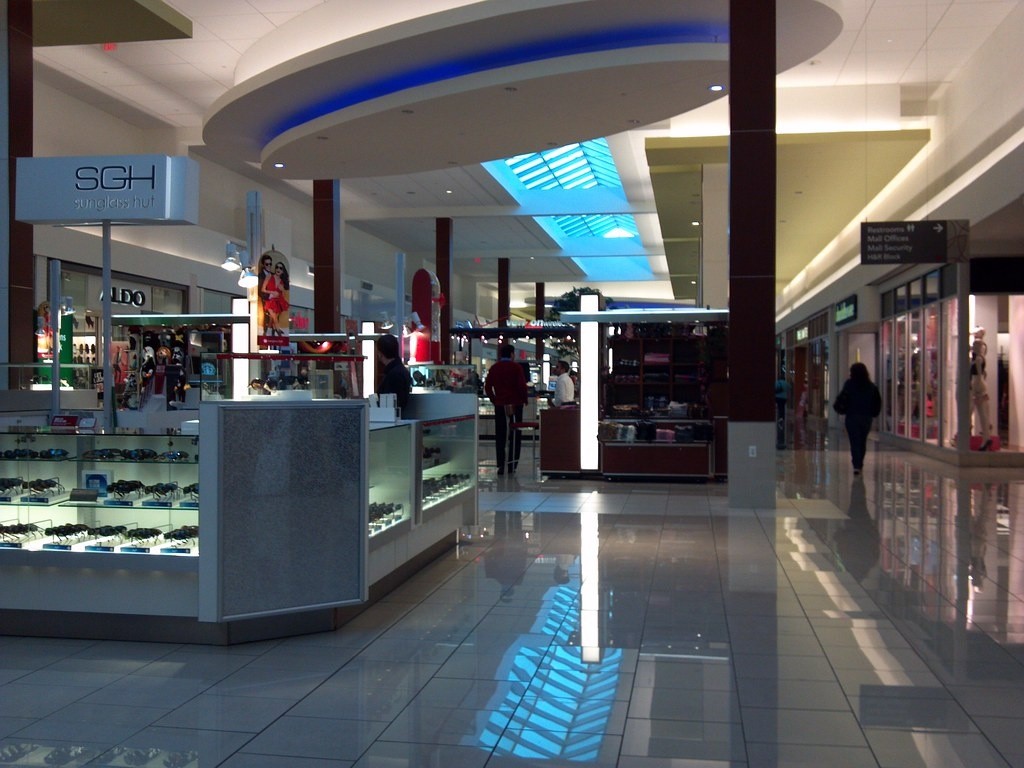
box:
[310,369,335,399]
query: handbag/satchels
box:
[775,380,784,393]
[834,379,852,415]
[597,401,714,443]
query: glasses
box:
[0,523,199,550]
[275,266,282,270]
[265,263,273,266]
[249,377,274,391]
[0,477,199,505]
[371,427,471,535]
[283,375,308,385]
[0,448,188,464]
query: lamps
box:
[379,311,394,330]
[60,296,74,317]
[34,316,48,337]
[220,242,241,272]
[237,250,259,289]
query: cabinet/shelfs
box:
[369,413,478,610]
[596,418,716,482]
[0,411,200,644]
[607,324,729,420]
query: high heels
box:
[978,440,992,451]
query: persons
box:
[259,255,290,336]
[485,346,528,475]
[777,375,792,419]
[834,363,881,475]
[554,361,577,408]
[377,335,410,416]
[970,327,993,451]
[466,372,484,396]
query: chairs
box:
[503,404,540,477]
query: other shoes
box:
[853,462,863,474]
[508,469,513,473]
[778,417,783,422]
[498,467,504,475]
